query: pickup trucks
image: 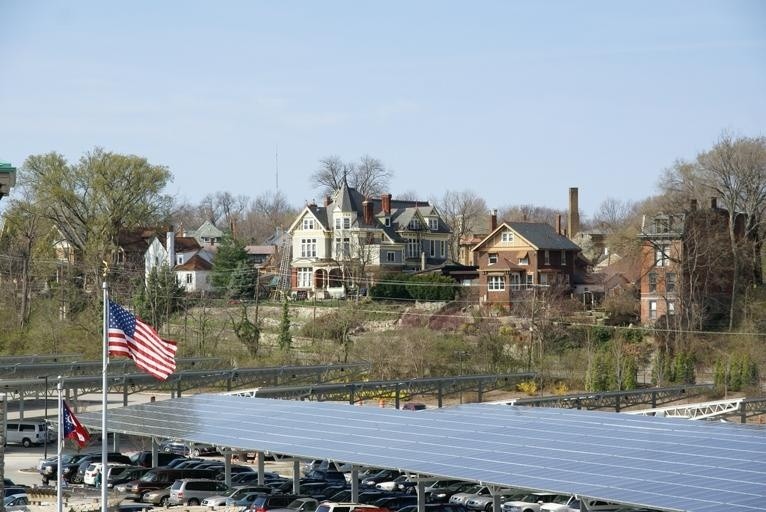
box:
[3,492,67,511]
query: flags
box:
[60,399,91,448]
[105,297,179,382]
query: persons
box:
[95,470,101,488]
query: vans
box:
[4,419,47,448]
